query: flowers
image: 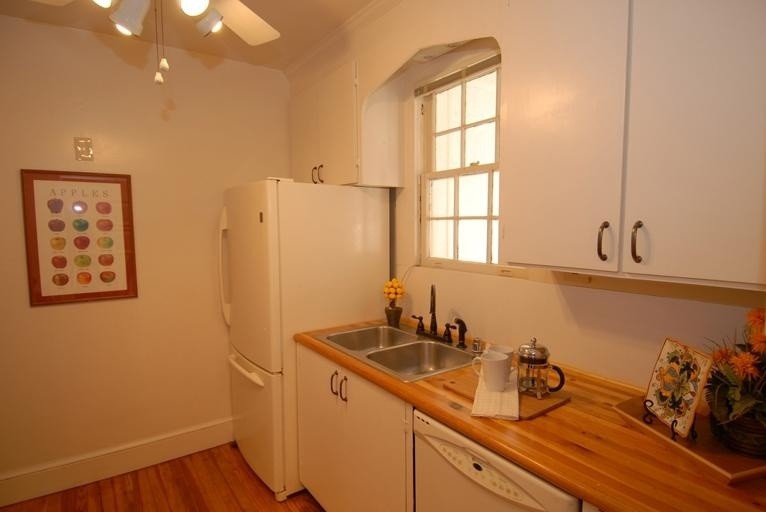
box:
[383,278,406,309]
[707,310,766,430]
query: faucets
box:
[453,318,467,350]
[430,284,438,336]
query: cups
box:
[488,345,515,382]
[472,353,510,392]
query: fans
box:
[34,0,281,47]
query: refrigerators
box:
[217,174,391,502]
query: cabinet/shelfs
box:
[288,61,404,186]
[496,1,766,290]
[294,342,407,511]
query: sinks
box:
[315,324,425,352]
[360,339,478,384]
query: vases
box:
[385,306,402,326]
[724,410,766,457]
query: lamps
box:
[92,0,223,84]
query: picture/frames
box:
[21,169,138,306]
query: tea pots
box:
[517,336,565,399]
